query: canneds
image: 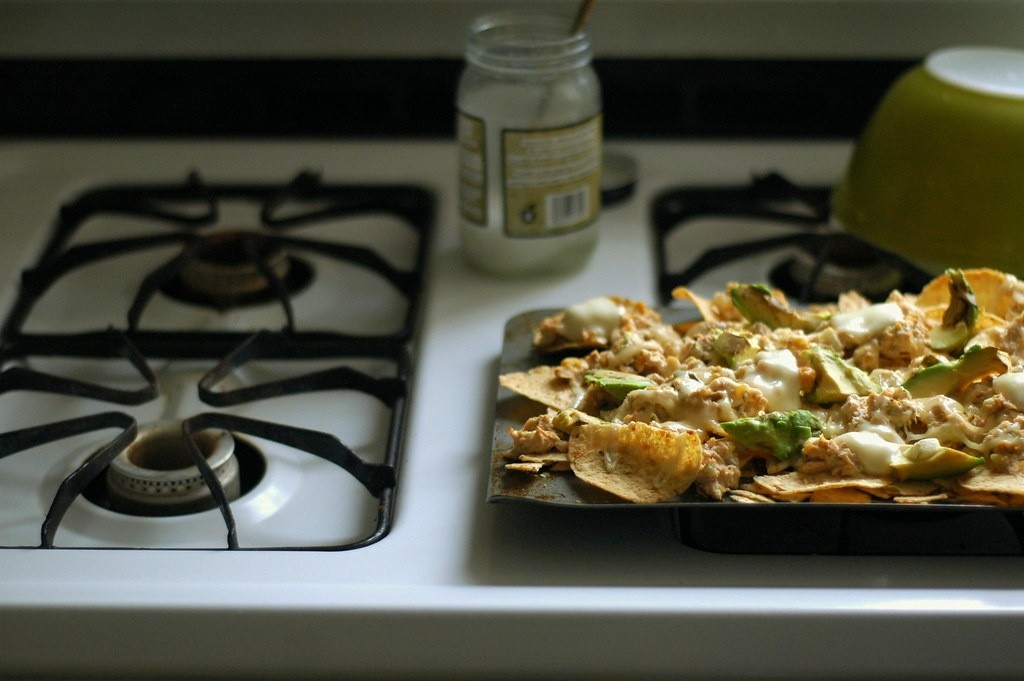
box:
[458,10,599,275]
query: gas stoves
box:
[0,137,1022,677]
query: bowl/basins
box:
[843,46,1024,283]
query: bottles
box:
[456,10,602,280]
